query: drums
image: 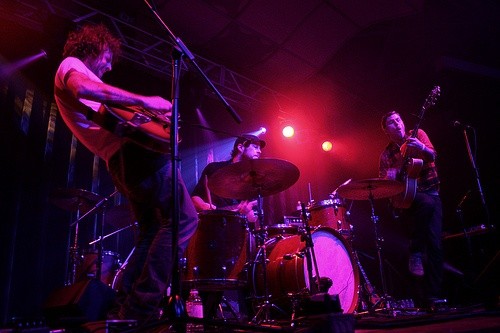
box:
[179,210,251,292]
[252,225,362,314]
[75,249,122,291]
[302,198,354,241]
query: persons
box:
[53,25,199,320]
[379,110,450,309]
[191,135,266,227]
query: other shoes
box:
[408,251,425,277]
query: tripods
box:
[366,189,406,316]
[248,190,290,326]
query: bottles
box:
[185,288,204,333]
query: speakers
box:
[39,278,116,323]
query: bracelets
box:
[420,144,425,152]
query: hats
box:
[234,135,265,149]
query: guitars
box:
[386,85,441,209]
[95,100,171,150]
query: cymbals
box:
[61,189,104,214]
[206,158,301,199]
[106,205,134,230]
[337,178,404,201]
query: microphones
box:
[452,118,461,129]
